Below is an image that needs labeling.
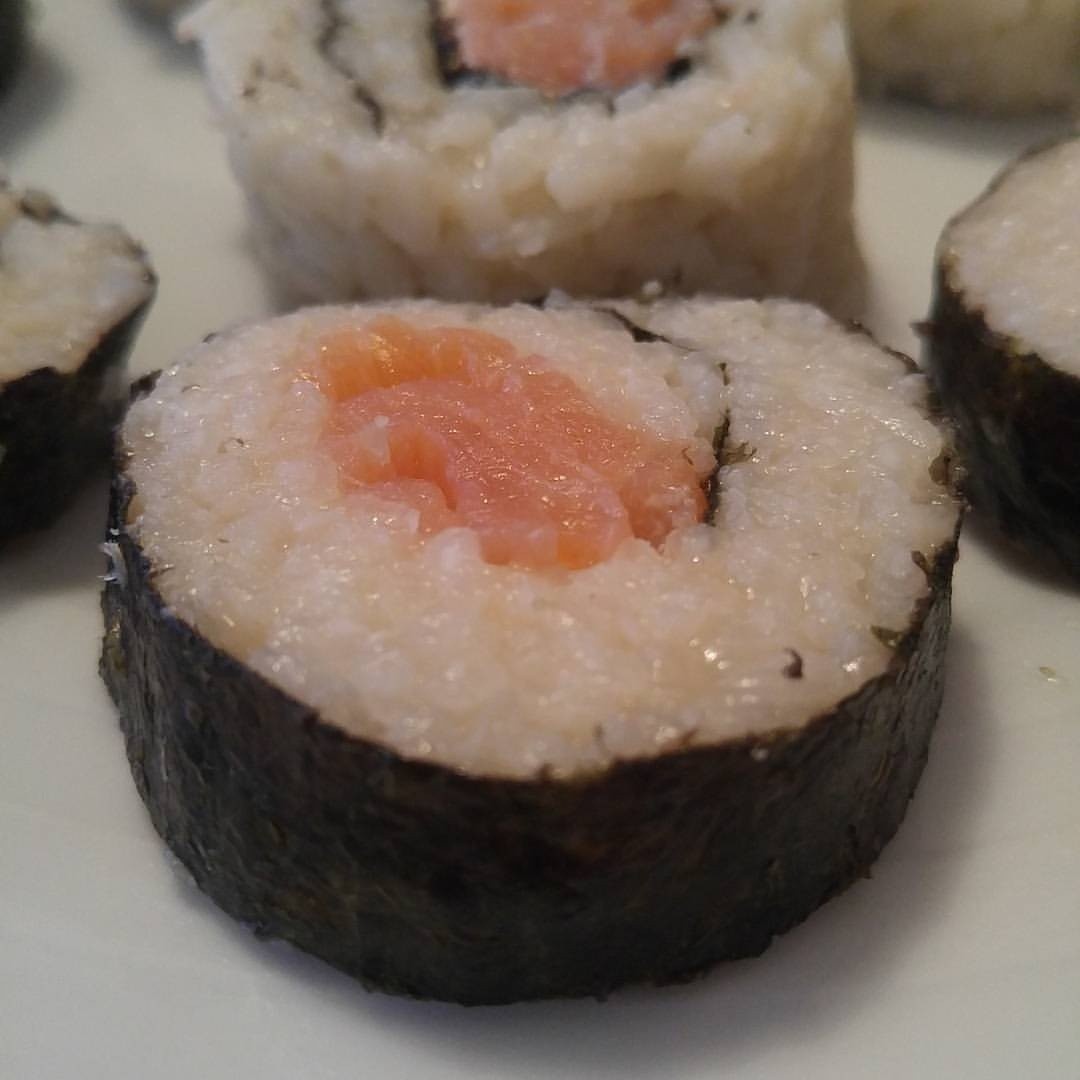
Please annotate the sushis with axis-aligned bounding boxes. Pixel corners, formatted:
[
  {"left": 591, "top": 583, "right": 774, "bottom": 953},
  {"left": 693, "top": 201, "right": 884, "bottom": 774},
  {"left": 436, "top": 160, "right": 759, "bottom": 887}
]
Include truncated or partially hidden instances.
[
  {"left": 840, "top": 1, "right": 1074, "bottom": 122},
  {"left": 914, "top": 132, "right": 1080, "bottom": 600},
  {"left": 76, "top": 268, "right": 976, "bottom": 1009},
  {"left": 175, "top": 1, "right": 867, "bottom": 322},
  {"left": 2, "top": 153, "right": 159, "bottom": 575}
]
